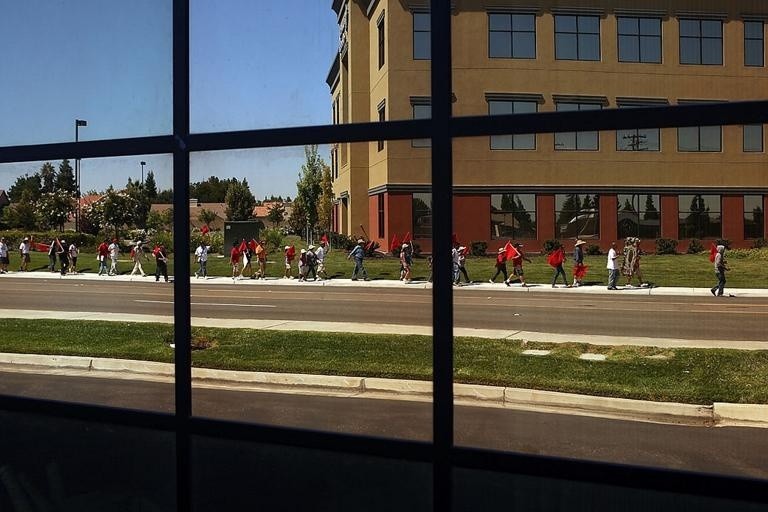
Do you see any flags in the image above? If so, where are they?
[502,243,516,260]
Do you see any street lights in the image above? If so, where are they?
[73,117,88,233]
[139,159,147,184]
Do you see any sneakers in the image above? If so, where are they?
[608,286,615,290]
[626,285,634,287]
[711,288,716,296]
[194,272,432,283]
[641,283,648,287]
[452,280,473,286]
[488,278,495,284]
[553,283,586,288]
[522,283,527,287]
[504,280,510,286]
[0,267,173,284]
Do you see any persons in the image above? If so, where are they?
[488,248,511,287]
[606,236,648,290]
[298,240,325,282]
[425,246,473,285]
[347,239,372,281]
[282,245,295,279]
[0,237,171,283]
[572,240,586,287]
[711,245,729,297]
[551,244,570,288]
[194,241,212,280]
[503,242,532,287]
[400,243,411,282]
[230,240,269,281]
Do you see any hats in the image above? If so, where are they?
[358,239,365,244]
[574,239,587,247]
[401,244,409,253]
[457,246,467,252]
[285,241,325,253]
[497,247,506,255]
